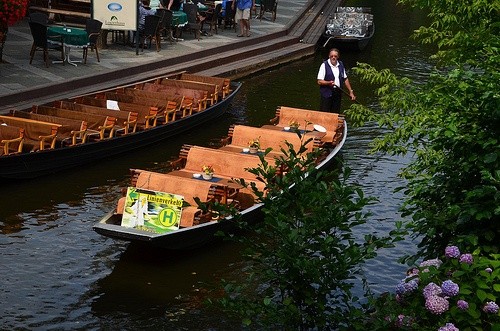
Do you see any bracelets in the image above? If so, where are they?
[349,89,353,93]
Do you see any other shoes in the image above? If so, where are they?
[246,32,251,37]
[237,33,244,37]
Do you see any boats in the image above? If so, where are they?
[0,73,244,183]
[93,106,348,248]
[321,7,375,60]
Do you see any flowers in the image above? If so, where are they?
[200,163,216,175]
[289,118,300,127]
[248,140,260,149]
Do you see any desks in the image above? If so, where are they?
[171,11,188,41]
[167,171,249,199]
[261,126,327,139]
[196,4,208,36]
[219,145,284,162]
[47,27,88,67]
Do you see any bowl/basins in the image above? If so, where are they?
[284,127,291,131]
[243,148,250,152]
[193,173,201,178]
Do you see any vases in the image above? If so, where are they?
[201,172,213,180]
[249,147,258,155]
[291,125,299,132]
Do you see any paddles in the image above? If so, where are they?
[335,84,361,104]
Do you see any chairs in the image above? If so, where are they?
[28,11,103,69]
[137,0,279,52]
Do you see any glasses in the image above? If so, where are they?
[330,55,338,58]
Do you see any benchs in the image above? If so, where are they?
[0,74,345,226]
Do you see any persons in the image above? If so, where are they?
[317,48,356,113]
[139,0,256,48]
